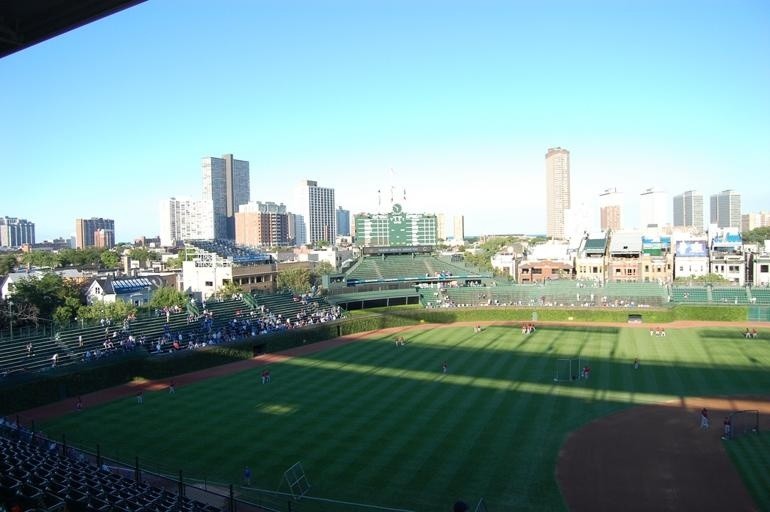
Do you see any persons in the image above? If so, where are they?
[701,408,711,427]
[661,326,665,336]
[583,366,592,378]
[634,357,641,371]
[0,296,224,443]
[424,269,651,307]
[478,323,482,332]
[395,336,398,348]
[751,328,760,339]
[521,321,536,334]
[241,463,250,485]
[655,325,660,337]
[442,360,450,373]
[650,325,654,336]
[743,327,752,339]
[723,415,731,441]
[401,336,405,347]
[261,368,271,384]
[224,284,342,342]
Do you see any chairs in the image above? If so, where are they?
[0,432,223,512]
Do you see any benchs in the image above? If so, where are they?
[342,253,494,281]
